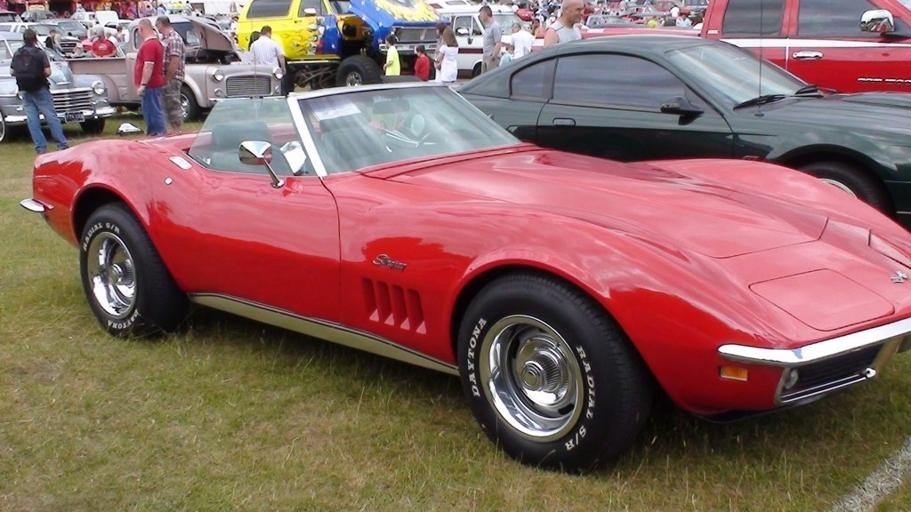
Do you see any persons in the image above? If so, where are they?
[383,0,708,84]
[10,29,70,154]
[155,15,186,132]
[134,19,169,136]
[250,26,287,96]
[46,0,239,59]
[246,31,260,63]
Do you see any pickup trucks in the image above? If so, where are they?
[59,10,269,125]
[579,1,909,95]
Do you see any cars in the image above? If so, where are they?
[453,33,881,210]
[1,34,112,140]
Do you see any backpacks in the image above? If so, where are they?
[14,48,45,92]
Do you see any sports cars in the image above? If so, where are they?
[19,83,911,476]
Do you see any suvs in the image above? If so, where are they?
[233,0,454,86]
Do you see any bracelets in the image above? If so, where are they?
[140,83,148,86]
